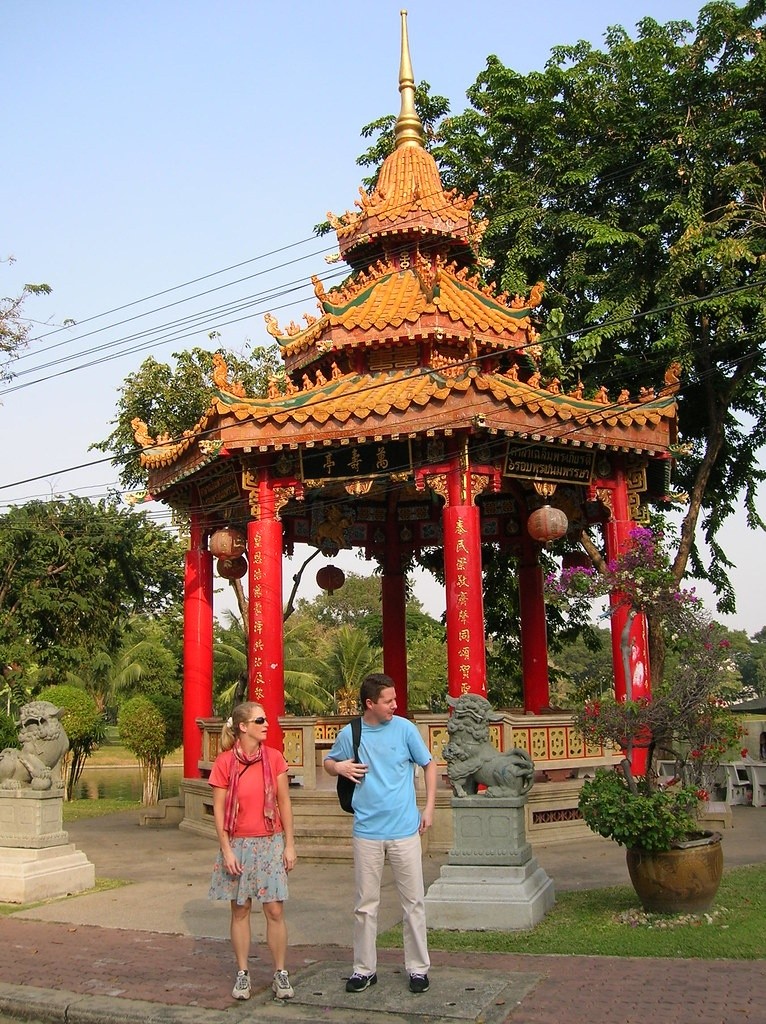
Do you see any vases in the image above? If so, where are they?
[620,830,724,914]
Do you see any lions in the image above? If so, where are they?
[0,699,71,791]
[441,692,536,798]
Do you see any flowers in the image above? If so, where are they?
[543,531,750,848]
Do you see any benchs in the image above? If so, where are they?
[314,738,337,767]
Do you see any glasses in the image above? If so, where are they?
[244,717,267,724]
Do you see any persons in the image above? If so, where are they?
[206,701,298,1000]
[323,673,436,993]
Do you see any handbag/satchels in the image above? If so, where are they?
[337,717,361,814]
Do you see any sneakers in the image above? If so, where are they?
[271,970,294,999]
[231,970,251,1000]
[410,974,430,992]
[346,972,377,992]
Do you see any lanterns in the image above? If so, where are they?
[528,507,566,546]
[217,556,247,586]
[210,529,245,568]
[562,551,591,569]
[316,565,345,596]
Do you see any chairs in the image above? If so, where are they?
[654,760,766,808]
